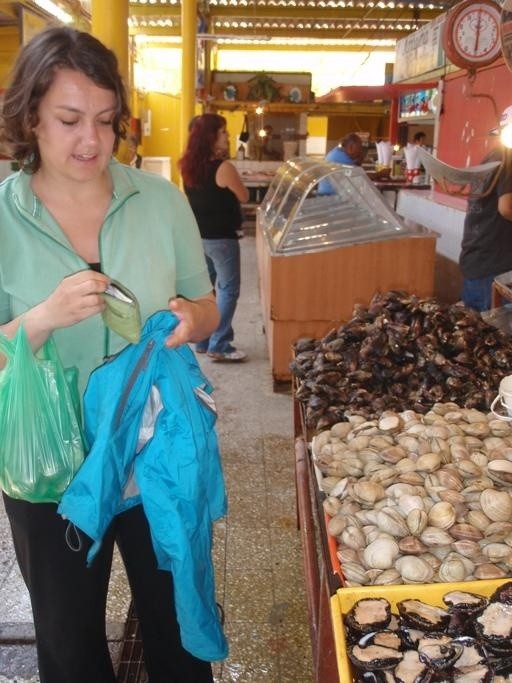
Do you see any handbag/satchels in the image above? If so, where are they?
[239,131,249,141]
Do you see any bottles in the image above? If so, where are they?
[399,102,430,118]
[236,143,245,160]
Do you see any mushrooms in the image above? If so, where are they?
[344,579,512,682]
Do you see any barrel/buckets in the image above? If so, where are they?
[490,374,512,427]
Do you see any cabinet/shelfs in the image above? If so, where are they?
[255,154,442,394]
[292,270,512,681]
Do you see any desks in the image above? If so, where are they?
[235,173,273,211]
[369,180,431,213]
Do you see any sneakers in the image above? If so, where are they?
[196,347,247,360]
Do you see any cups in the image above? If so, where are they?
[405,167,421,184]
[374,161,390,181]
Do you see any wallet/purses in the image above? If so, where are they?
[95,279,142,344]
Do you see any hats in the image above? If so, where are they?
[488,105,512,137]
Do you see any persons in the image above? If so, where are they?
[318,134,362,196]
[0,27,220,683]
[237,125,280,161]
[180,114,250,360]
[413,131,427,152]
[459,106,512,314]
